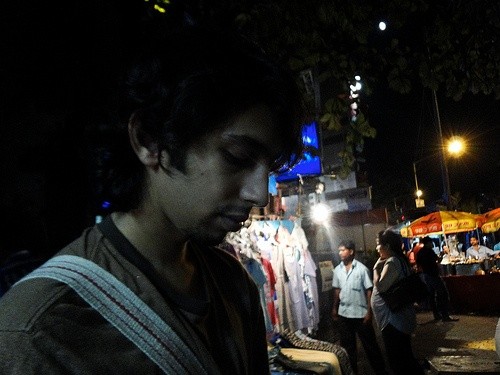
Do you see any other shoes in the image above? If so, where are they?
[432,315,460,322]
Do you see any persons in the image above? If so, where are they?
[0,49,304,375]
[332,228,459,375]
[465,237,500,261]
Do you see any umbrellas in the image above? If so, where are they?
[401,208,500,244]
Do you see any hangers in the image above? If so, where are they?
[226,215,300,235]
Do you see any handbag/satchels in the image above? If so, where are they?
[379,269,429,314]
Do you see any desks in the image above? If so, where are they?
[439,272,500,316]
